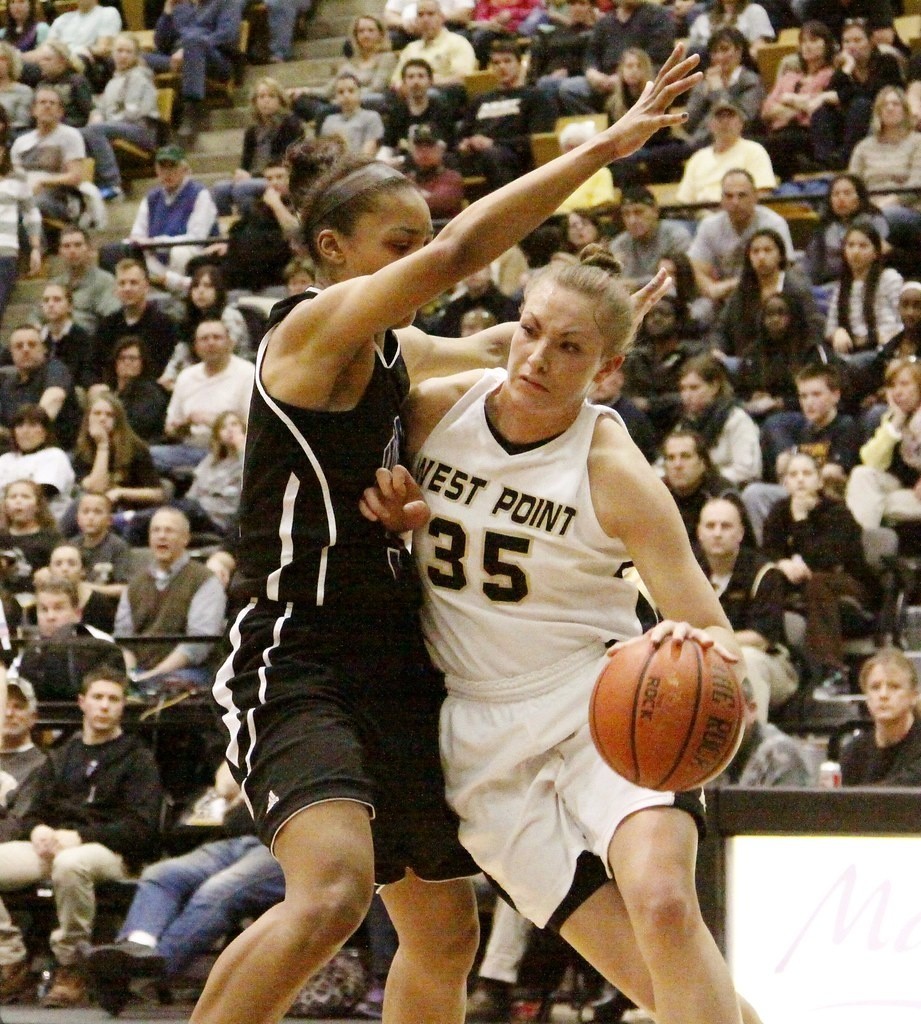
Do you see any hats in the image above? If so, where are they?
[3,673,36,703]
[709,94,742,114]
[47,39,70,60]
[414,124,439,147]
[156,145,185,162]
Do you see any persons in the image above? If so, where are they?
[0,0,921,1024]
[188,39,703,1023]
[0,668,163,1009]
[835,649,921,792]
[361,242,761,1024]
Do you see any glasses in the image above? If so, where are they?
[118,354,138,363]
[844,18,866,27]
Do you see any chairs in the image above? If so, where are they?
[39,156,96,230]
[106,87,174,177]
[154,19,250,108]
[247,3,308,37]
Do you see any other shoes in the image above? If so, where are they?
[1,942,644,1024]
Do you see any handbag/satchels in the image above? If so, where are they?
[293,946,364,1014]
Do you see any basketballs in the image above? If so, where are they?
[582,630,749,797]
